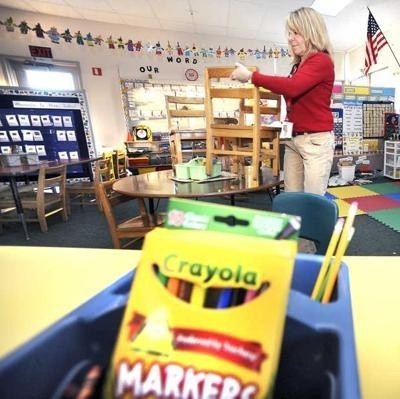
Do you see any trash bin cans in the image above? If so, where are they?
[337,163,356,181]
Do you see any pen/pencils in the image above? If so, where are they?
[108,199,358,399]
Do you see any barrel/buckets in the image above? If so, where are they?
[339,164,356,180]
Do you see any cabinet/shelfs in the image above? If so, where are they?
[383,140,400,180]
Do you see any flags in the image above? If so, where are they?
[364,13,388,76]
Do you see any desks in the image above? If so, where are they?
[0,244,400,399]
[112,165,284,215]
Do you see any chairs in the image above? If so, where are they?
[163,66,282,181]
[0,149,168,250]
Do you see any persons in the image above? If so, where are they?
[230,7,336,197]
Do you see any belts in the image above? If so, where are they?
[291,128,319,137]
